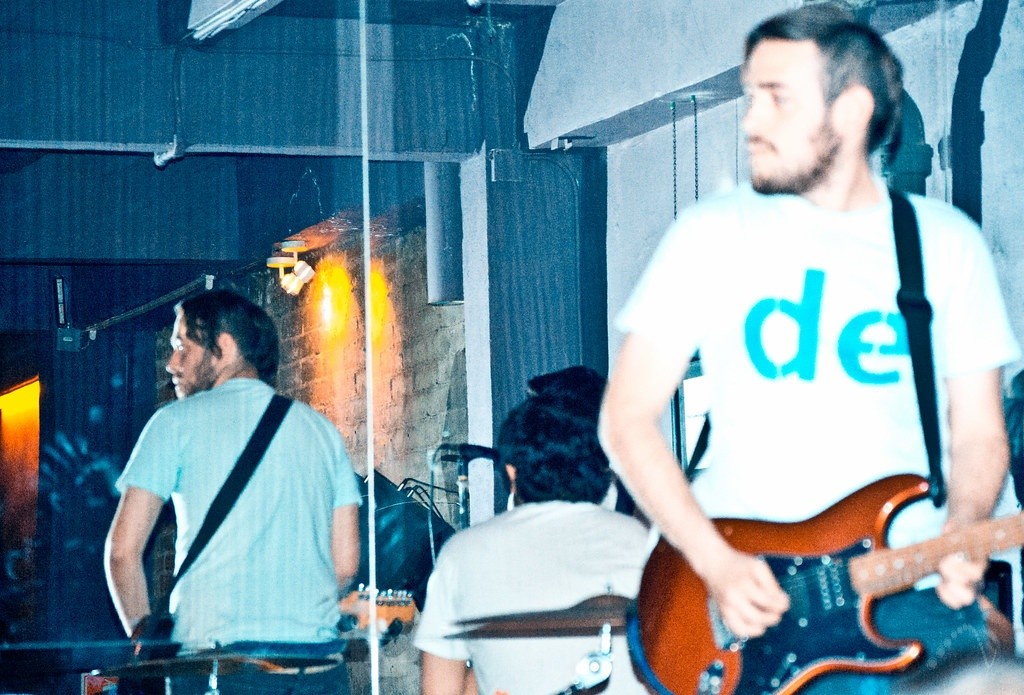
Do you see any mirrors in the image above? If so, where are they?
[0,145,494,695]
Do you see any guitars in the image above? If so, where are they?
[79,582,416,695]
[628,468,1024,695]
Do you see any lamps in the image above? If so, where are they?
[268,241,315,297]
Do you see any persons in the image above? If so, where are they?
[596,5,1023,695]
[103,286,364,695]
[414,365,658,695]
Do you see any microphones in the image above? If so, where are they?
[442,443,498,460]
